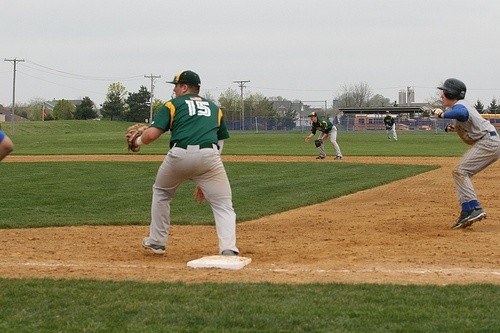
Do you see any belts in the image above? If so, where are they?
[175,142,213,149]
[480,130,497,139]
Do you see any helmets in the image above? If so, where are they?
[438,78,466,99]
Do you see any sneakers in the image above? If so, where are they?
[461,206,486,222]
[141,237,166,254]
[452,210,472,229]
[222,250,236,256]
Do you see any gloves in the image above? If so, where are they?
[431,108,444,119]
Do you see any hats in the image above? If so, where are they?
[308,111,317,117]
[386,111,389,113]
[166,70,201,87]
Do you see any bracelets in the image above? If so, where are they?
[136,136,144,146]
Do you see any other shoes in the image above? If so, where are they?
[335,156,342,159]
[316,156,325,160]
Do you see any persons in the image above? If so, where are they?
[126,71,241,256]
[302,111,344,160]
[383,116,398,142]
[430,77,500,229]
[0,130,14,159]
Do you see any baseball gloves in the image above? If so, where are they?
[385,123,392,130]
[444,123,457,133]
[126,123,149,153]
[314,139,324,148]
